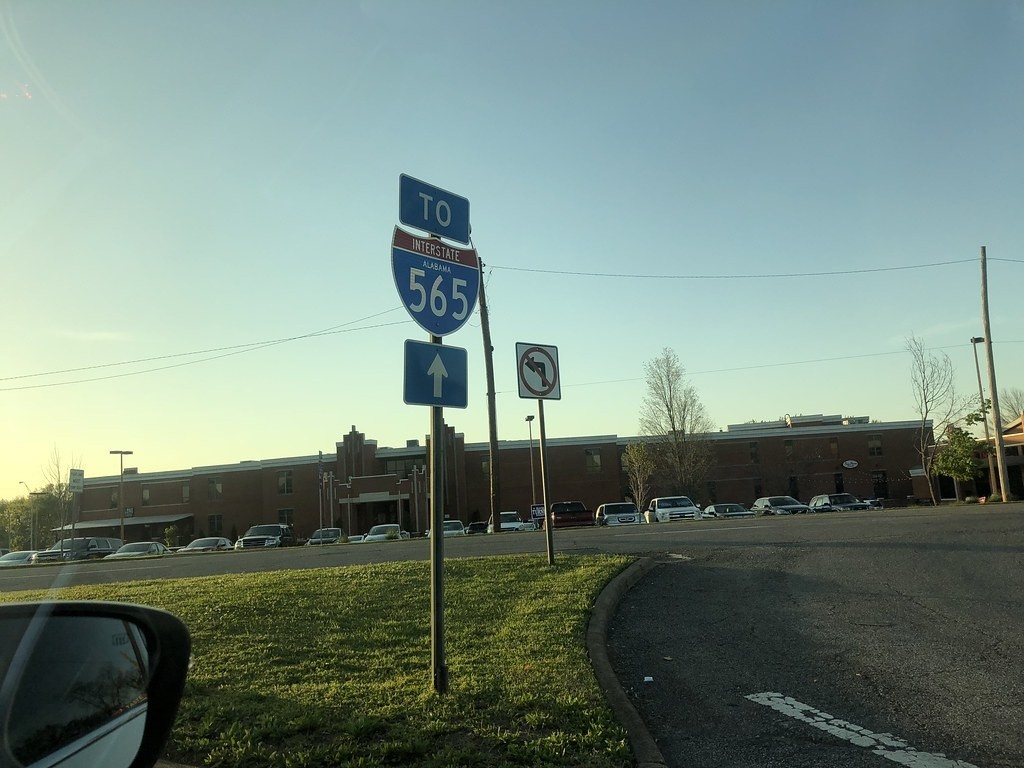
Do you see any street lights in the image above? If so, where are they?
[970,336,996,497]
[525,415,535,530]
[110,450,134,542]
[18,481,34,551]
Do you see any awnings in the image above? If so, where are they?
[50,513,192,531]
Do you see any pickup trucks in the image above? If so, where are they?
[541,501,596,530]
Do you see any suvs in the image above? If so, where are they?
[642,496,703,523]
[233,523,298,550]
[30,537,125,564]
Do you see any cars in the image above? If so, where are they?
[176,537,234,552]
[307,528,348,545]
[102,542,172,559]
[347,535,365,544]
[522,523,537,531]
[424,519,467,538]
[594,502,647,526]
[483,511,528,534]
[808,493,872,513]
[0,551,42,566]
[702,504,756,519]
[857,499,884,510]
[747,495,811,517]
[362,524,407,541]
[465,521,487,534]
